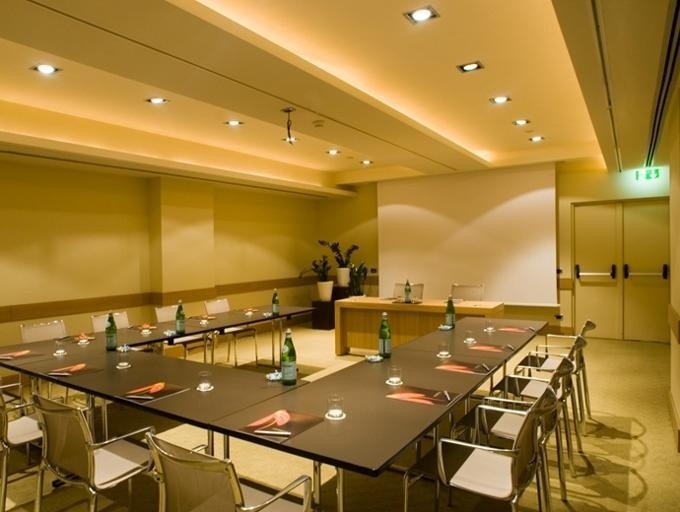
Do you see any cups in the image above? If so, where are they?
[484,318,496,330]
[77,331,89,343]
[438,342,450,356]
[117,352,132,369]
[141,326,151,335]
[326,393,345,419]
[464,328,475,342]
[197,369,211,389]
[55,341,64,354]
[199,319,208,328]
[387,365,402,383]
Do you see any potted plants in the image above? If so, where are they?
[311,256,335,301]
[347,260,368,299]
[319,240,359,286]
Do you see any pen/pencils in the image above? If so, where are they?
[507,344,517,351]
[529,327,535,331]
[126,396,154,399]
[482,363,491,372]
[0,356,14,360]
[129,324,134,330]
[253,430,292,436]
[444,390,451,401]
[57,334,70,342]
[48,372,72,376]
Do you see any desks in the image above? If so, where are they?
[2,304,316,370]
[332,293,506,358]
[13,348,309,462]
[206,317,550,512]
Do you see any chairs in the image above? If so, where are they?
[28,388,160,512]
[204,296,259,368]
[18,318,69,399]
[447,283,487,301]
[400,386,550,512]
[1,381,66,512]
[392,282,425,301]
[153,305,202,360]
[449,319,595,500]
[145,431,315,511]
[90,310,130,333]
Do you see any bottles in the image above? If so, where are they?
[404,279,411,304]
[271,287,279,315]
[378,312,391,359]
[104,312,118,350]
[174,299,185,335]
[280,328,297,387]
[445,295,455,328]
